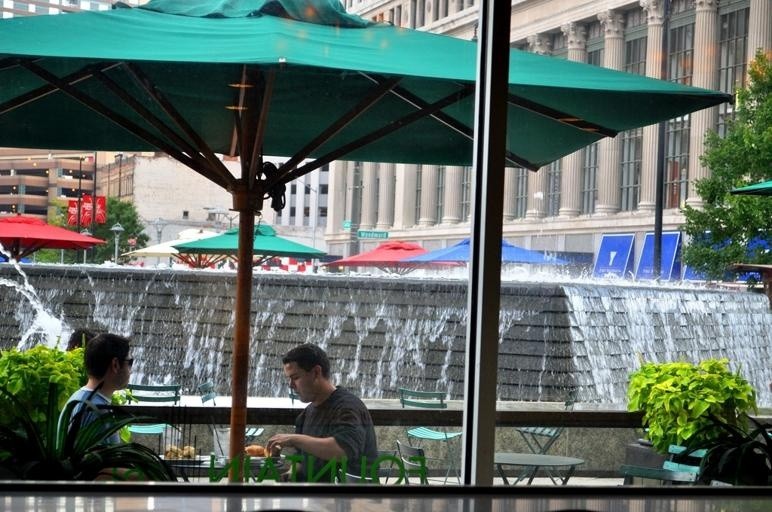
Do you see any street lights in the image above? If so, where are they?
[80,229,94,264]
[77,155,85,233]
[114,153,123,198]
[111,222,125,266]
[153,217,167,266]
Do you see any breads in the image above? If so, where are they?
[265,446,281,457]
[183,446,196,458]
[246,446,264,455]
[165,446,181,459]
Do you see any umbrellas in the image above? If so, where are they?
[320,237,465,280]
[0,1,737,510]
[0,214,105,267]
[117,225,232,269]
[397,235,570,273]
[171,220,329,272]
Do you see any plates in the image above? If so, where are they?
[158,456,205,466]
[242,453,286,461]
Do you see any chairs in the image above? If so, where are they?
[514,386,579,485]
[126,381,183,455]
[197,377,265,455]
[397,385,464,484]
[337,468,376,485]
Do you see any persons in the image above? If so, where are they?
[51,334,151,482]
[261,340,379,482]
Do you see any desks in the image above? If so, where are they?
[494,453,586,487]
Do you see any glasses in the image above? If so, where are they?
[123,359,134,367]
